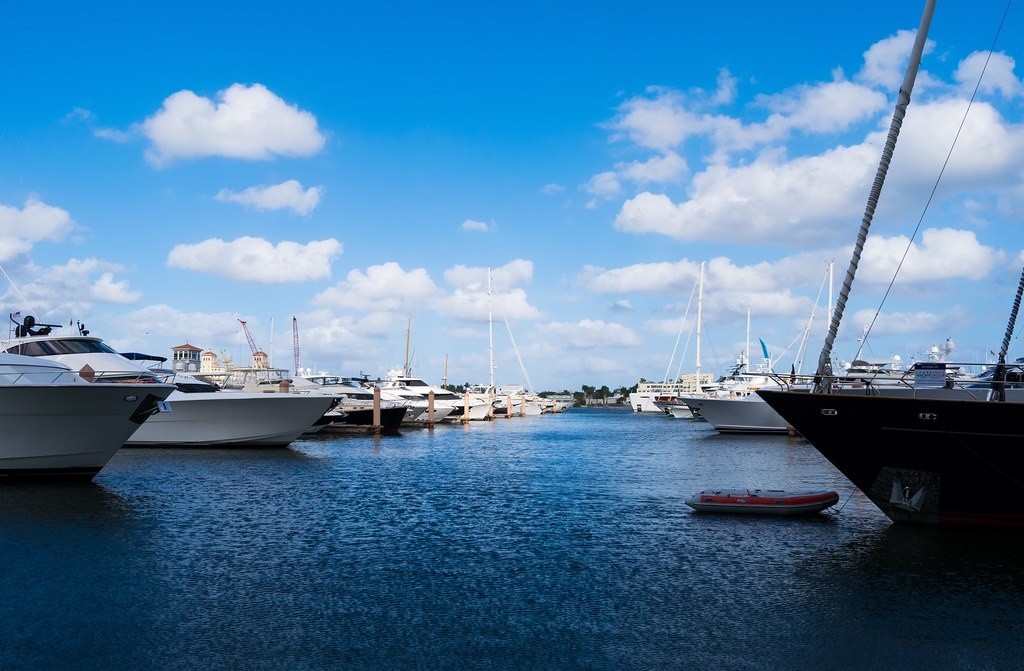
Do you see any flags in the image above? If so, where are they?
[759,338,771,361]
[990,350,995,357]
[12,312,21,318]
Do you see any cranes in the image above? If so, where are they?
[292,316,302,379]
[233,317,270,377]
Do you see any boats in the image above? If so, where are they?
[651,261,789,419]
[674,259,1024,435]
[220,366,575,433]
[755,1,1024,556]
[122,352,344,449]
[0,266,178,481]
[684,485,842,516]
